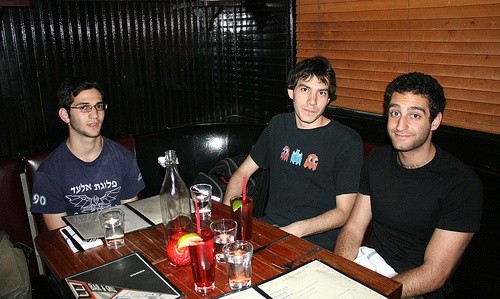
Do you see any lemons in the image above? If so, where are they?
[178,233,203,247]
[233,200,242,212]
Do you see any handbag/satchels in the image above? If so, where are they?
[0,229,32,299]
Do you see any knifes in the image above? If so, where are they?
[253,233,292,254]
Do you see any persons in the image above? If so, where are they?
[0,230,63,299]
[333,72,483,299]
[30,78,146,230]
[222,55,365,253]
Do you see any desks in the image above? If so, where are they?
[33,191,402,299]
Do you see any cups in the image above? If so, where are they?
[189,226,216,297]
[222,240,253,290]
[190,184,213,221]
[230,195,252,241]
[100,208,125,249]
[210,219,237,264]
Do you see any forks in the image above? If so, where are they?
[272,247,322,270]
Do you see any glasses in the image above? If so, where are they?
[70,103,108,111]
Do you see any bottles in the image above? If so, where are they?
[159,150,195,267]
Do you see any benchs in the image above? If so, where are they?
[1,124,500,299]
